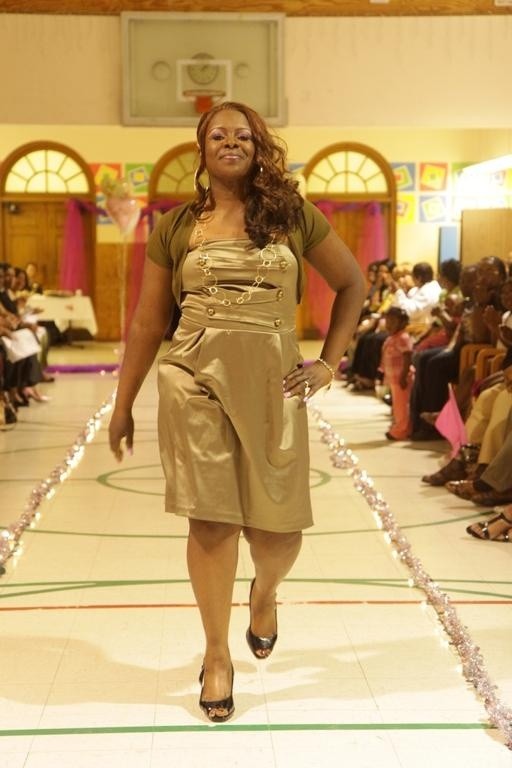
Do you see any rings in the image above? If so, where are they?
[304,380,310,396]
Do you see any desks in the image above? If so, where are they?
[18,292,97,349]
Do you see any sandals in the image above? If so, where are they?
[198,654,236,723]
[493,529,512,542]
[466,512,511,540]
[246,575,277,659]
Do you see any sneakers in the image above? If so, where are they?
[349,381,376,394]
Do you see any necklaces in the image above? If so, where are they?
[191,211,276,311]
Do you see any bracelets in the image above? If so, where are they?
[317,357,334,390]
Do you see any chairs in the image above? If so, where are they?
[458,342,507,389]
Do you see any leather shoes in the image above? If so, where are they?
[422,475,430,482]
[430,459,466,484]
[445,479,477,500]
[41,376,54,382]
[472,487,512,505]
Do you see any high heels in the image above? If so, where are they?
[12,398,29,412]
[22,386,51,402]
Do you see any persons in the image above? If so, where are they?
[1,259,59,433]
[105,100,368,722]
[342,257,512,542]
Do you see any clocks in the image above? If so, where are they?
[189,54,219,84]
